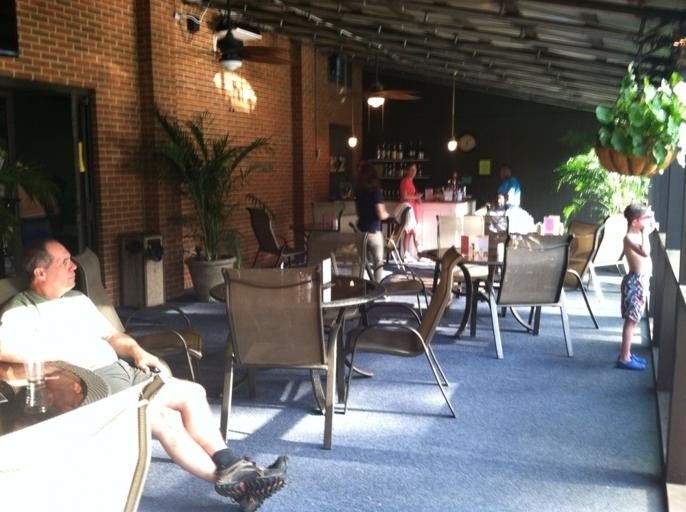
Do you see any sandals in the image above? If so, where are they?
[617,353,647,370]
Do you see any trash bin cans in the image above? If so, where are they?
[118,231,165,308]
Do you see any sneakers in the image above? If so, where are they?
[214,455,290,511]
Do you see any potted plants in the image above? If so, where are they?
[121,105,277,304]
[592,59,686,176]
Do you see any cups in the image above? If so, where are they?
[297,270,313,289]
[298,290,312,304]
[24,347,45,383]
[25,383,47,415]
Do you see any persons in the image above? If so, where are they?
[398,163,424,262]
[617,204,652,371]
[496,164,522,208]
[354,163,390,283]
[0,238,289,511]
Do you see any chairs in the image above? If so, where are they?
[0,275,31,312]
[70,246,205,382]
[0,372,166,512]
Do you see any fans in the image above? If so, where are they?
[333,52,425,103]
[185,0,291,66]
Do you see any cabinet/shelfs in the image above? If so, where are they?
[366,158,433,200]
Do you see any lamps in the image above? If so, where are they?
[218,59,244,112]
[366,97,386,134]
[215,21,263,42]
[447,74,459,152]
[347,95,358,149]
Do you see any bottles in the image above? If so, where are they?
[375,139,426,198]
[434,171,472,200]
[329,153,347,172]
